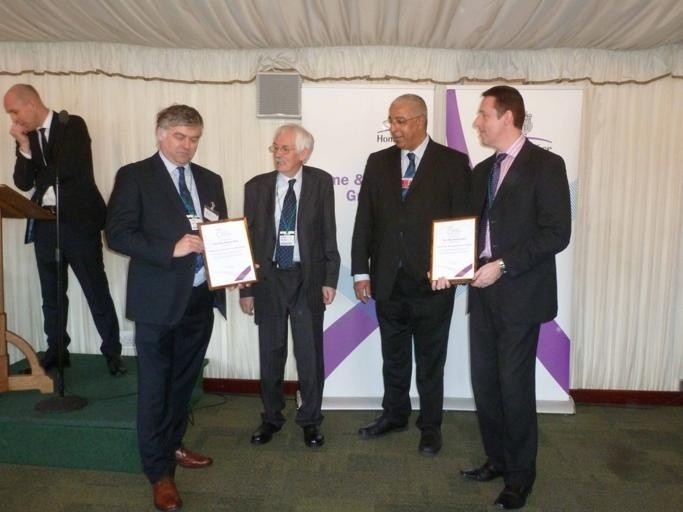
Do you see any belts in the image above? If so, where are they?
[41,206,60,215]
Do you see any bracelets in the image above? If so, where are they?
[496,255,508,275]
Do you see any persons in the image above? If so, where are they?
[239,122,340,448]
[349,92,472,457]
[3,83,128,380]
[97,104,228,510]
[428,85,573,508]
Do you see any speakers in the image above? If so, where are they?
[257,71,301,119]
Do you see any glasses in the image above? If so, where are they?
[383,116,420,126]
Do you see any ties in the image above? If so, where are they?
[175,166,205,273]
[400,152,417,205]
[38,127,62,205]
[474,154,509,260]
[275,179,298,271]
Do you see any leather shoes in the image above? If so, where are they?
[359,413,409,439]
[175,446,212,468]
[460,457,502,482]
[419,424,443,456]
[40,346,71,368]
[104,354,130,376]
[153,477,183,511]
[250,421,281,445]
[494,483,533,510]
[303,424,324,448]
